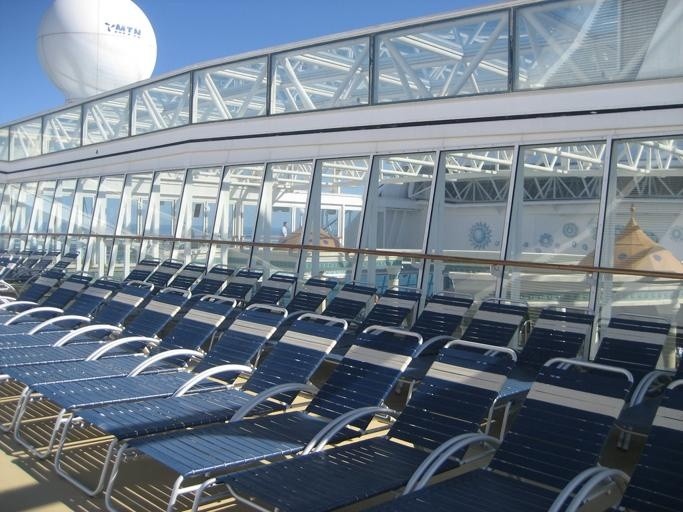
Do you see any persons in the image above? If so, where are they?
[281,221,289,239]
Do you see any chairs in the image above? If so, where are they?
[1,249,681,510]
